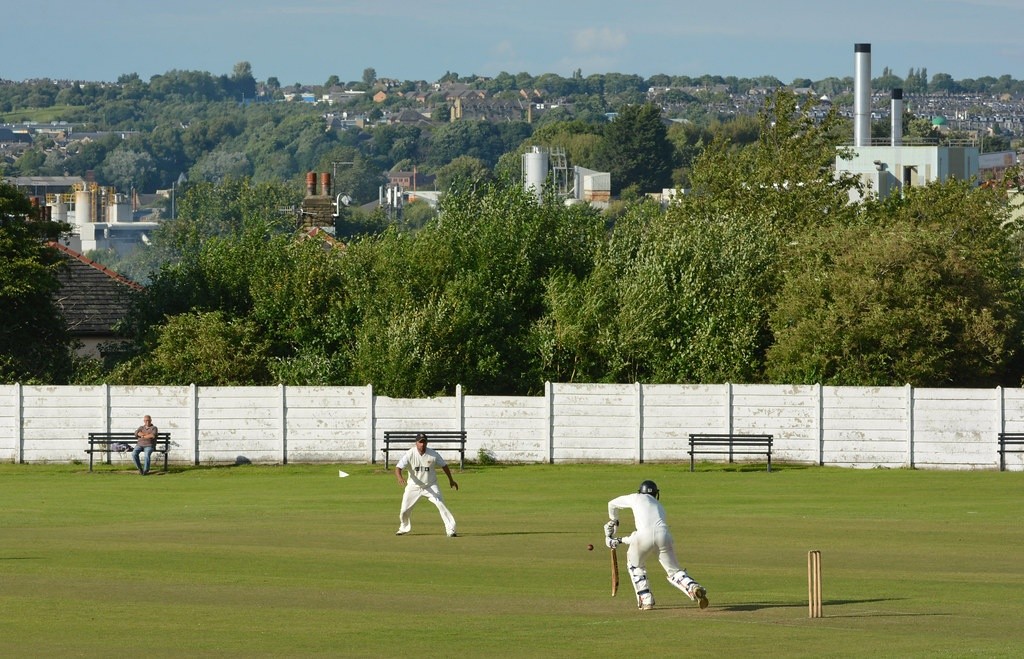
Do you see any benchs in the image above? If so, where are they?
[380,431,468,469]
[84,433,172,471]
[997,433,1024,471]
[687,433,774,472]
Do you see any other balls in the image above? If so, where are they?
[587,544,594,550]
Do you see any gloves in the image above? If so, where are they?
[610,538,621,548]
[604,522,615,537]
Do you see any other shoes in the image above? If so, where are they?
[142,471,146,475]
[447,533,456,538]
[138,469,143,475]
[396,531,406,535]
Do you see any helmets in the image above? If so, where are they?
[639,481,657,498]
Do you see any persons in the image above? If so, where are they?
[604,480,709,610]
[395,433,459,537]
[132,415,158,476]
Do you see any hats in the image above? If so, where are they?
[416,434,427,442]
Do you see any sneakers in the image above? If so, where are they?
[639,604,653,610]
[693,586,709,610]
